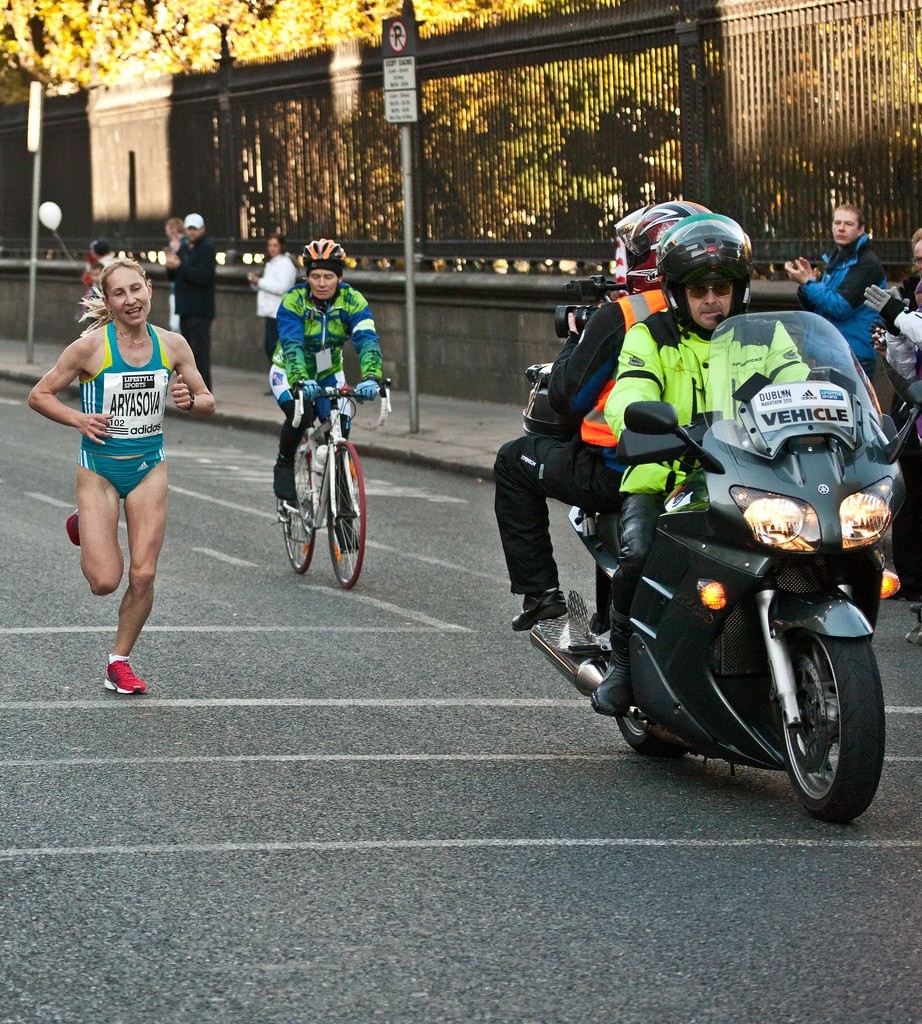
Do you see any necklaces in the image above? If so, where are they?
[118,330,149,344]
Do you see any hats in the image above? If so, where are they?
[185,213,204,229]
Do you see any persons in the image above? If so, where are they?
[81,240,113,331]
[248,235,299,397]
[267,239,382,550]
[28,258,216,694]
[162,212,217,396]
[493,198,850,716]
[863,228,922,645]
[784,205,887,391]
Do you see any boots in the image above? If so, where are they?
[591,604,633,717]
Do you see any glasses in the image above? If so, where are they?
[685,281,731,298]
[912,256,920,265]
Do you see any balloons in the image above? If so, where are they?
[39,201,62,230]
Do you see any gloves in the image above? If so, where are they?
[294,379,321,400]
[863,285,910,314]
[869,319,888,345]
[356,380,379,405]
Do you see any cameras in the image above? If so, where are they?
[878,331,888,346]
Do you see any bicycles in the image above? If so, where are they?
[275,376,393,590]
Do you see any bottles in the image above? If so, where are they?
[314,445,327,488]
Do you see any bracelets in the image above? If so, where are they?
[185,392,194,410]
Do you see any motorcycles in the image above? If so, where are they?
[525,309,921,826]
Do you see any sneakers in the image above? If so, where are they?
[274,454,297,501]
[512,588,567,631]
[65,509,80,546]
[104,660,147,694]
[335,518,360,550]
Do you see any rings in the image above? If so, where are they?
[180,390,182,396]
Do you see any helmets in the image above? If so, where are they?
[625,200,713,295]
[302,238,347,276]
[656,213,752,325]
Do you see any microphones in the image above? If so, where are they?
[562,280,605,300]
[716,314,727,324]
